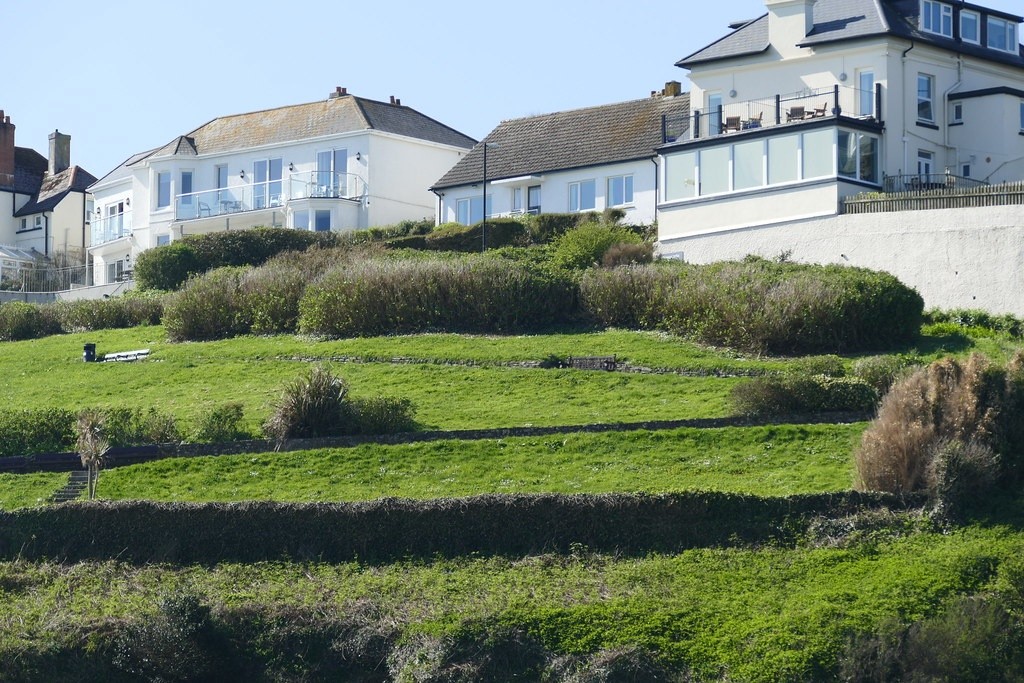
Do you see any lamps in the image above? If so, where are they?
[356,152,360,160]
[288,163,293,170]
[240,170,244,178]
[96,207,100,214]
[126,198,130,205]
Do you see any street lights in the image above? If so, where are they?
[479,141,500,251]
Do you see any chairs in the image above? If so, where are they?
[786,103,827,124]
[198,201,211,217]
[721,111,763,133]
[229,201,241,212]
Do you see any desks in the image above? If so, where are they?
[217,199,233,214]
